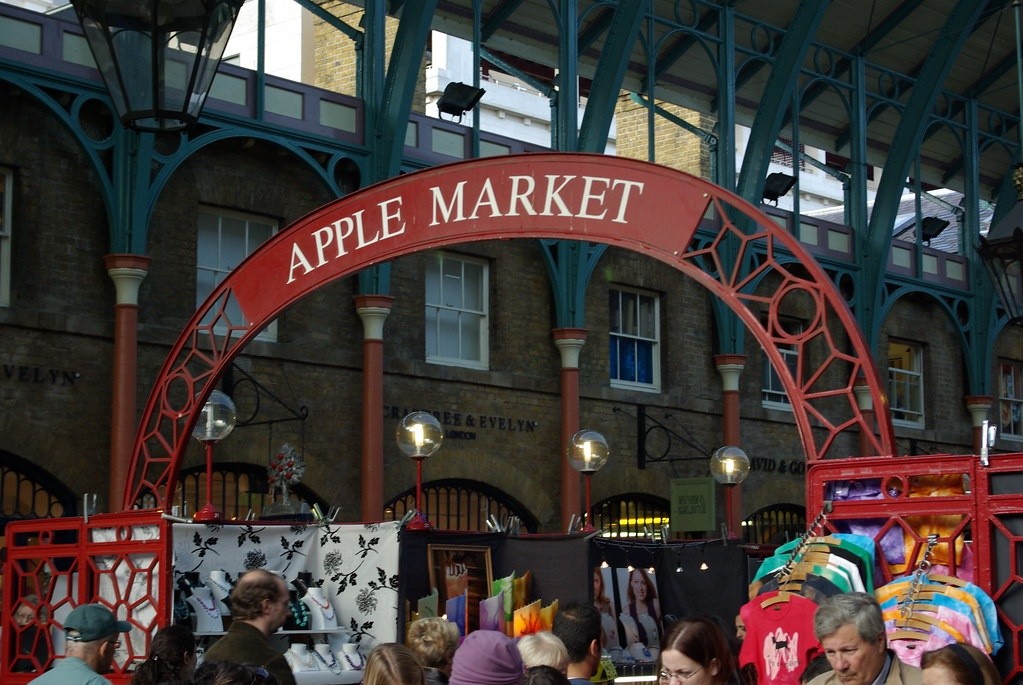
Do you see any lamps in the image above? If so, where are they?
[913,216,951,249]
[599,540,709,574]
[437,81,486,126]
[762,173,799,208]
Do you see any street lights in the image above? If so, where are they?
[710,446,750,539]
[183,389,237,522]
[394,411,444,530]
[566,429,609,533]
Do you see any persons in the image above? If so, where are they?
[132,626,197,685]
[193,569,297,685]
[620,569,661,623]
[593,568,615,622]
[920,643,1001,685]
[735,613,757,685]
[799,593,921,685]
[655,616,741,685]
[363,600,604,685]
[27,603,132,685]
[11,594,54,673]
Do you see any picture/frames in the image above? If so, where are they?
[427,544,493,631]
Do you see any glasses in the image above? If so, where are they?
[100,640,121,648]
[191,650,203,658]
[660,665,704,682]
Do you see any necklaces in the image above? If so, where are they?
[288,648,315,668]
[289,598,307,626]
[602,614,615,639]
[298,579,317,591]
[313,650,343,675]
[312,596,335,620]
[345,651,364,670]
[641,615,657,639]
[174,600,190,620]
[620,618,638,643]
[194,596,220,619]
[208,577,232,594]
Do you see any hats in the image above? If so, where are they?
[64,604,133,642]
[449,630,522,685]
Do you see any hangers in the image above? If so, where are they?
[888,551,973,645]
[778,507,841,597]
